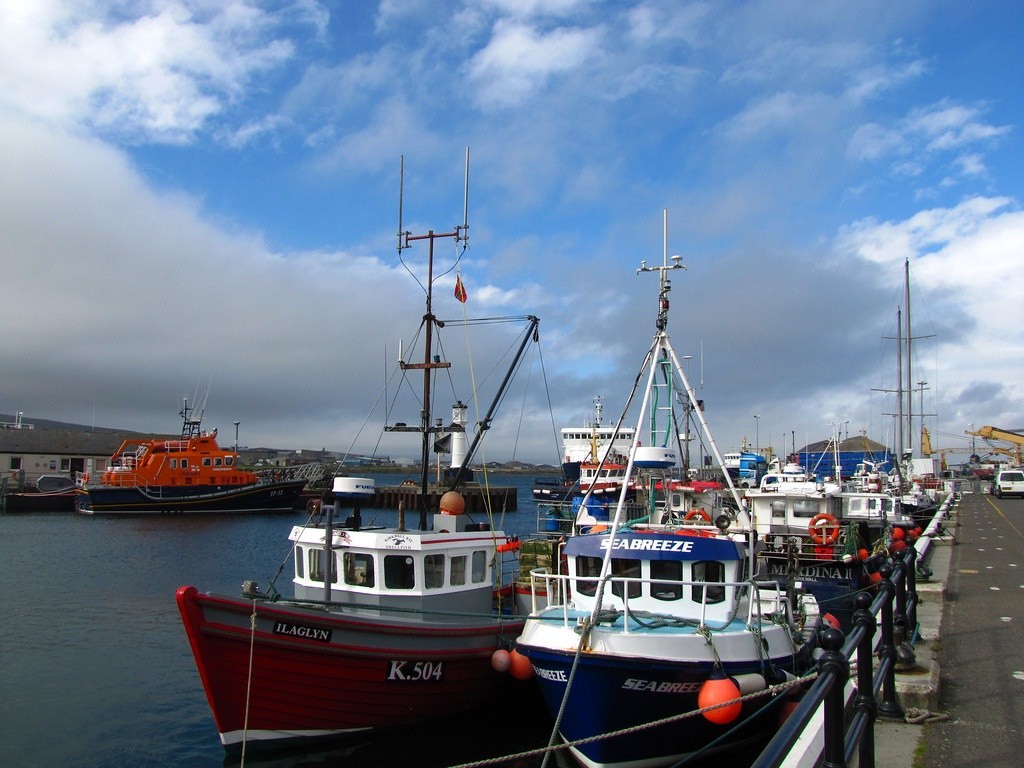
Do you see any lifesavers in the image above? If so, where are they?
[83,472,89,484]
[809,514,840,544]
[684,509,711,525]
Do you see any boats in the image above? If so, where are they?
[73,398,322,517]
[510,204,826,768]
[533,391,945,558]
[174,141,553,762]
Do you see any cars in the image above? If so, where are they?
[990,471,1024,499]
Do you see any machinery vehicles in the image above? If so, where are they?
[964,426,1024,464]
